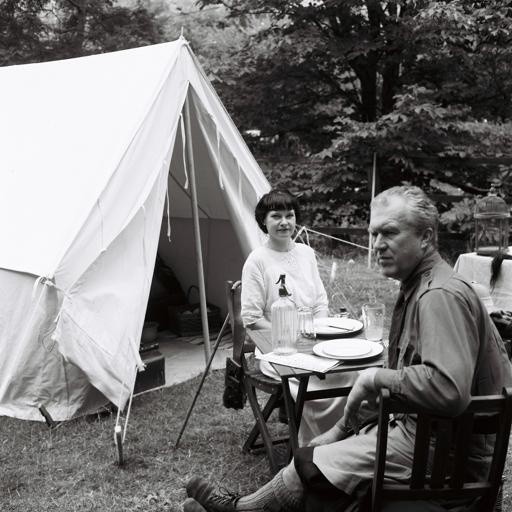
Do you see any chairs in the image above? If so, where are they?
[293,384,512,511]
[225,277,286,476]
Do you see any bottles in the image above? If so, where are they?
[270,273,299,356]
[335,307,352,318]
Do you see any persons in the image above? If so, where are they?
[240,188,363,450]
[180,184,511,512]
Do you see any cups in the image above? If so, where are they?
[361,303,386,341]
[297,307,317,346]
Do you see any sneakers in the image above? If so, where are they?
[273,421,292,469]
[181,474,246,512]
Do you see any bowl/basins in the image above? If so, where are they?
[140,322,159,343]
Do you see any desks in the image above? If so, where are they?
[458,246,512,317]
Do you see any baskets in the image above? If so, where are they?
[168,285,222,337]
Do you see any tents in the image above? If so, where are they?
[0,36,273,421]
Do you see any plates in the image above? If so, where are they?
[301,318,384,362]
